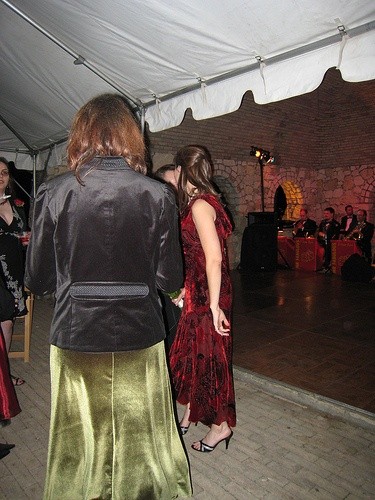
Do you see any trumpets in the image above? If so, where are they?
[292,218,304,236]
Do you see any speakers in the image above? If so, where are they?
[247,211,278,272]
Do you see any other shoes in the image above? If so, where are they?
[0,443,15,460]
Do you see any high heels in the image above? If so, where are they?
[179,421,199,435]
[191,430,233,452]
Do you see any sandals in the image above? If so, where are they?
[10,374,25,386]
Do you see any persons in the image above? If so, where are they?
[293,208,317,238]
[167,145,237,451]
[25,94,193,497]
[1,157,27,386]
[318,208,340,268]
[153,162,182,361]
[356,209,373,263]
[340,205,357,239]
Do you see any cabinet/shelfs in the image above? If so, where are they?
[293,238,325,273]
[331,240,362,275]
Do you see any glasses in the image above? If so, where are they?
[0,169,10,177]
[300,214,305,217]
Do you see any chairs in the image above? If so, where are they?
[8,286,34,363]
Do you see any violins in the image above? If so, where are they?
[346,221,367,238]
